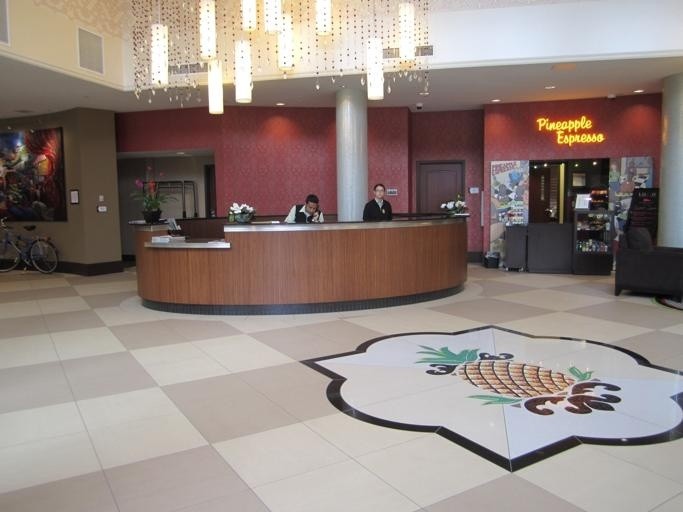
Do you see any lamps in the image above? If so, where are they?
[131,0,429,114]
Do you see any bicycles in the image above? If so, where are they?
[0,216,59,273]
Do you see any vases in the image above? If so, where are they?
[234,213,253,224]
[141,211,161,223]
[447,211,456,218]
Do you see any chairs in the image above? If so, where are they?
[616,234,683,296]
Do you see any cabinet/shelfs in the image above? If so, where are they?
[572,209,614,274]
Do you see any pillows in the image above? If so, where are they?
[629,226,653,248]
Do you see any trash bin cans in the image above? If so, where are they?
[484,251,500,268]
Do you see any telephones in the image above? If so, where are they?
[307,208,318,222]
[152,235,173,244]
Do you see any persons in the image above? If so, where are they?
[285,194,325,223]
[363,184,393,222]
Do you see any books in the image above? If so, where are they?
[151,234,186,243]
[208,239,225,244]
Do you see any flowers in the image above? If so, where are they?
[229,202,256,215]
[440,200,467,210]
[129,163,178,210]
